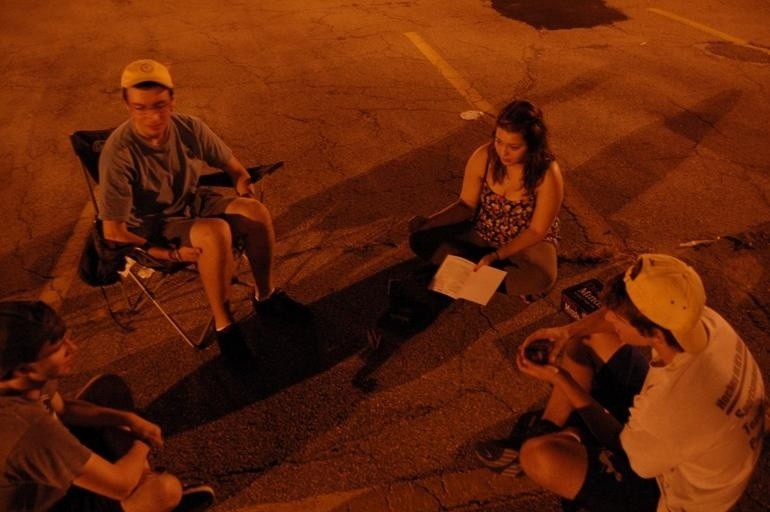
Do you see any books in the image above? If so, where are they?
[426,253,508,305]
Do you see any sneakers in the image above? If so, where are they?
[251,287,312,320]
[471,431,539,480]
[182,480,216,511]
[214,322,250,380]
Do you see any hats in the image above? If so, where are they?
[120,59,174,91]
[623,253,709,354]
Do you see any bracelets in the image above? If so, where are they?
[166,248,182,264]
[495,251,500,260]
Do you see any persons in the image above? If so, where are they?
[0,298,218,510]
[95,58,316,382]
[408,96,567,298]
[517,248,766,510]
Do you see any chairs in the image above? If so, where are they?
[69,128,286,353]
